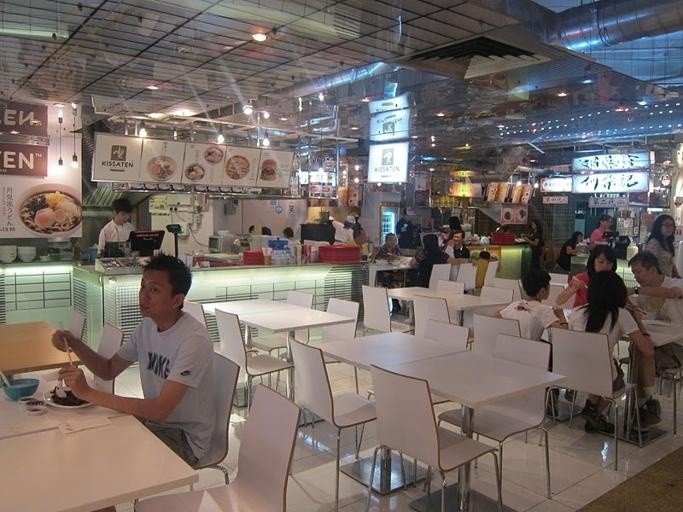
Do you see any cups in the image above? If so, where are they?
[263,255,272,265]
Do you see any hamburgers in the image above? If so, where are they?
[261,159,278,180]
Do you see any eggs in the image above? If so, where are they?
[54,209,66,222]
[34,208,56,227]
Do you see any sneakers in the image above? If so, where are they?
[580,398,616,434]
[565,389,577,404]
[546,388,560,417]
[630,395,664,433]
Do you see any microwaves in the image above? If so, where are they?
[207,235,234,253]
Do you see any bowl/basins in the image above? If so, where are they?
[39,255,48,261]
[0,245,37,264]
[145,146,250,181]
[3,378,39,399]
[14,184,82,236]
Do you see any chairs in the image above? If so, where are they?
[0,215,683,511]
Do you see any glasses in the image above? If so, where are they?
[660,222,675,228]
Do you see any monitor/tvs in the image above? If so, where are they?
[129,230,165,257]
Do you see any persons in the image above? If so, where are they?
[96,196,135,259]
[52,250,217,512]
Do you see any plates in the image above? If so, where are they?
[18,396,46,415]
[502,207,527,223]
[43,388,95,408]
[647,321,676,331]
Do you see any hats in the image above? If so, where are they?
[346,215,356,224]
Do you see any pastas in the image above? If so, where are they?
[21,192,83,231]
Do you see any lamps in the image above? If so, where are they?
[56,117,63,166]
[262,127,270,147]
[137,119,147,137]
[217,102,224,144]
[71,107,78,163]
